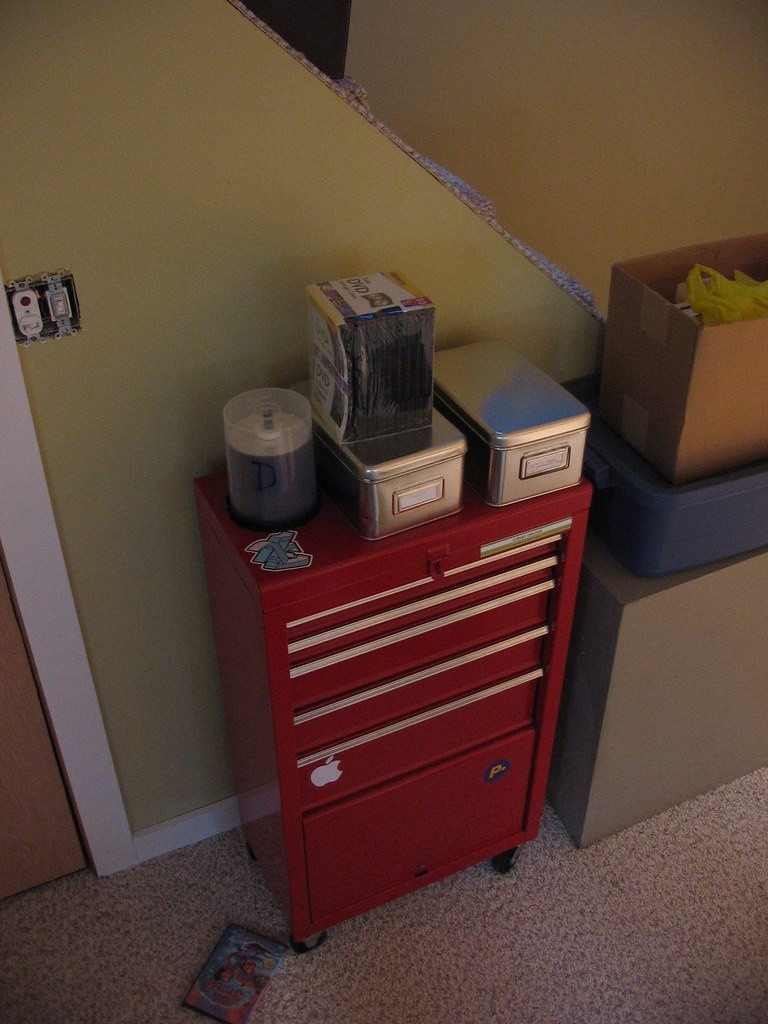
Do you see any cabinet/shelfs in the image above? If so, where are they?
[192,465,591,957]
[547,526,768,849]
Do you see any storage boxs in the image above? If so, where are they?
[562,372,768,578]
[601,232,768,485]
[308,407,466,541]
[304,269,436,447]
[435,337,589,506]
